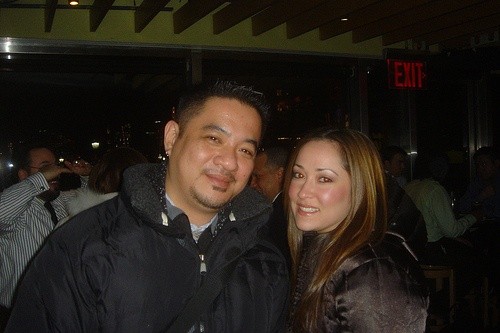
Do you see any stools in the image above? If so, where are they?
[407,264,456,333]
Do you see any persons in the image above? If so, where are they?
[281,126,430,333]
[54,145,149,228]
[402,140,485,321]
[455,146,500,282]
[249,146,295,237]
[380,145,410,224]
[0,140,95,332]
[7,80,291,333]
